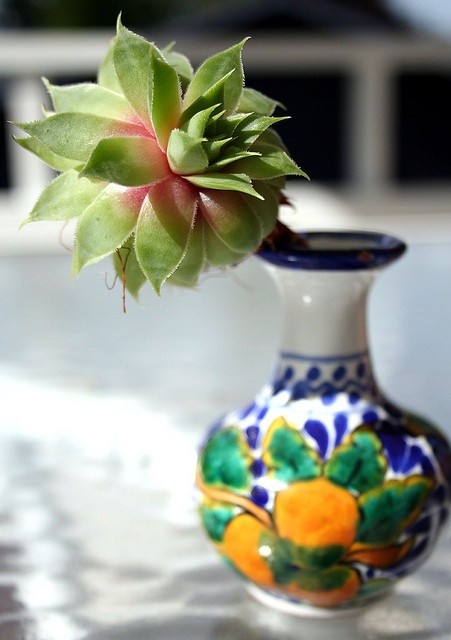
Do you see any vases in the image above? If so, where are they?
[194,228,451,619]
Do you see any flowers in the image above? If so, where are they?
[5,11,314,292]
